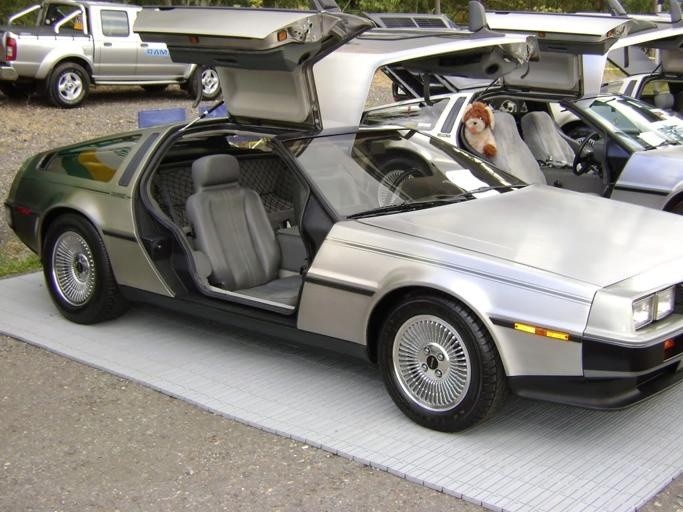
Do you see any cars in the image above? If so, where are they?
[1,0,221,109]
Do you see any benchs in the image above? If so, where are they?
[149,158,296,236]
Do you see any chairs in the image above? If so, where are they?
[654,93,683,120]
[490,111,549,187]
[186,154,304,307]
[520,110,583,174]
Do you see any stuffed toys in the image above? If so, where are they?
[461,100,496,159]
